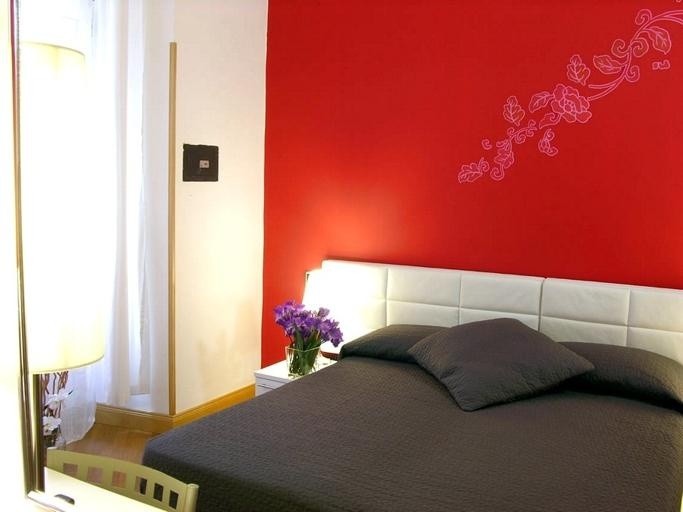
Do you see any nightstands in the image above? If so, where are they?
[255,357,338,397]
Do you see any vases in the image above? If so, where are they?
[283,344,321,375]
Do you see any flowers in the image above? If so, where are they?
[272,300,343,375]
[43,388,73,448]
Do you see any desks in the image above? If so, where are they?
[43,464,168,512]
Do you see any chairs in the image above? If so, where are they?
[45,447,199,512]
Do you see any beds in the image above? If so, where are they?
[139,260,683,512]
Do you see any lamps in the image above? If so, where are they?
[18,43,109,505]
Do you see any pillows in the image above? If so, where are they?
[406,319,595,411]
[557,330,682,417]
[338,324,451,364]
